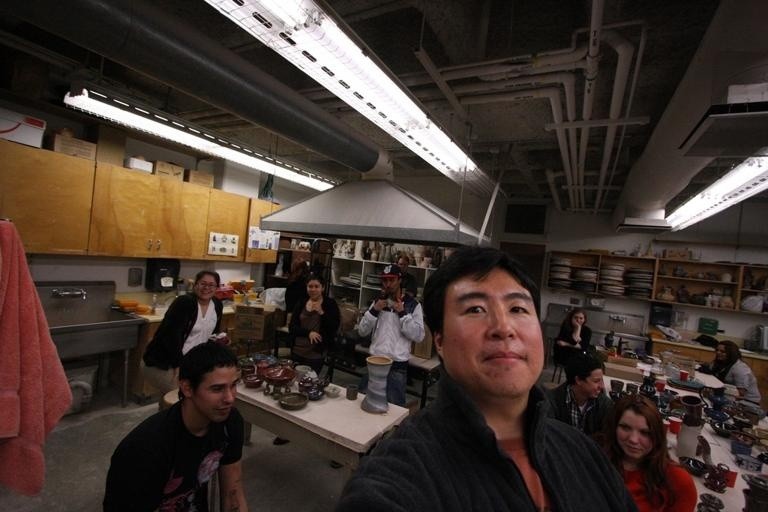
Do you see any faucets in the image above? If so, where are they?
[80,288,88,304]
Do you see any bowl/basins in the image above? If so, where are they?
[703,400,768,473]
[610,380,638,393]
[243,374,263,387]
[679,456,705,477]
[324,386,341,397]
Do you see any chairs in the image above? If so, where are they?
[550,336,565,384]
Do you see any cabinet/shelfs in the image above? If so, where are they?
[88,161,210,260]
[0,139,96,255]
[329,257,437,310]
[547,250,768,315]
[203,188,282,264]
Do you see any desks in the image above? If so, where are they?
[233,379,409,480]
[603,358,768,512]
[274,327,442,409]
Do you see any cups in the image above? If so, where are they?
[680,370,688,380]
[241,366,255,375]
[656,382,664,391]
[669,416,681,431]
[726,469,738,487]
[347,384,359,400]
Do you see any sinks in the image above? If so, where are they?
[541,302,649,353]
[33,281,140,361]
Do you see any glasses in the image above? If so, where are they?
[196,281,218,289]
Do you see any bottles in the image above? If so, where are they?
[657,263,736,309]
[360,355,394,413]
[332,237,443,268]
[289,237,314,252]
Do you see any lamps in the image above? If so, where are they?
[64,88,342,192]
[205,0,506,200]
[665,157,768,232]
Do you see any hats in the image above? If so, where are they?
[379,264,404,279]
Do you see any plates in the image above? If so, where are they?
[295,364,311,372]
[551,253,654,299]
[280,393,308,409]
[264,367,295,383]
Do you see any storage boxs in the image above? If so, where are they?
[0,109,214,188]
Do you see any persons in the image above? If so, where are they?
[546,353,612,437]
[101,342,249,511]
[600,390,700,511]
[140,269,225,410]
[331,245,638,510]
[555,307,594,381]
[273,234,426,470]
[706,341,763,406]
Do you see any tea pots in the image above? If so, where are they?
[299,377,313,391]
